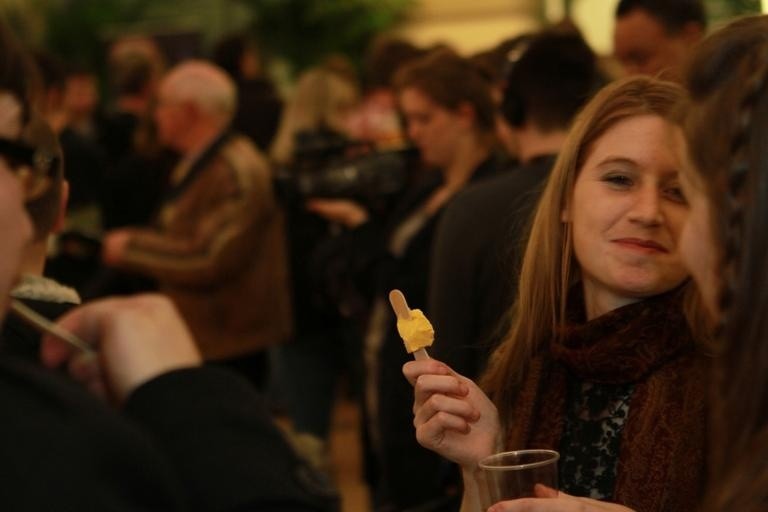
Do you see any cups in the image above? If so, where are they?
[476,448,561,512]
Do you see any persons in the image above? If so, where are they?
[2,0,768,512]
